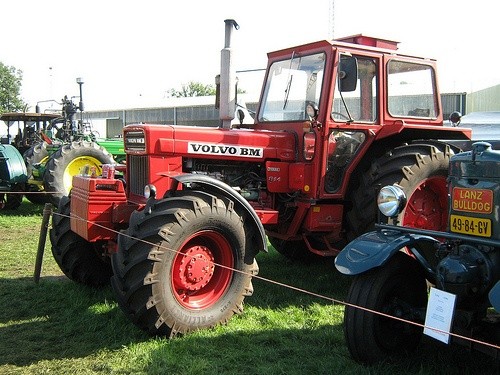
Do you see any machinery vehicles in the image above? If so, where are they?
[48,19,475,336]
[333,140,500,362]
[1,78,115,213]
